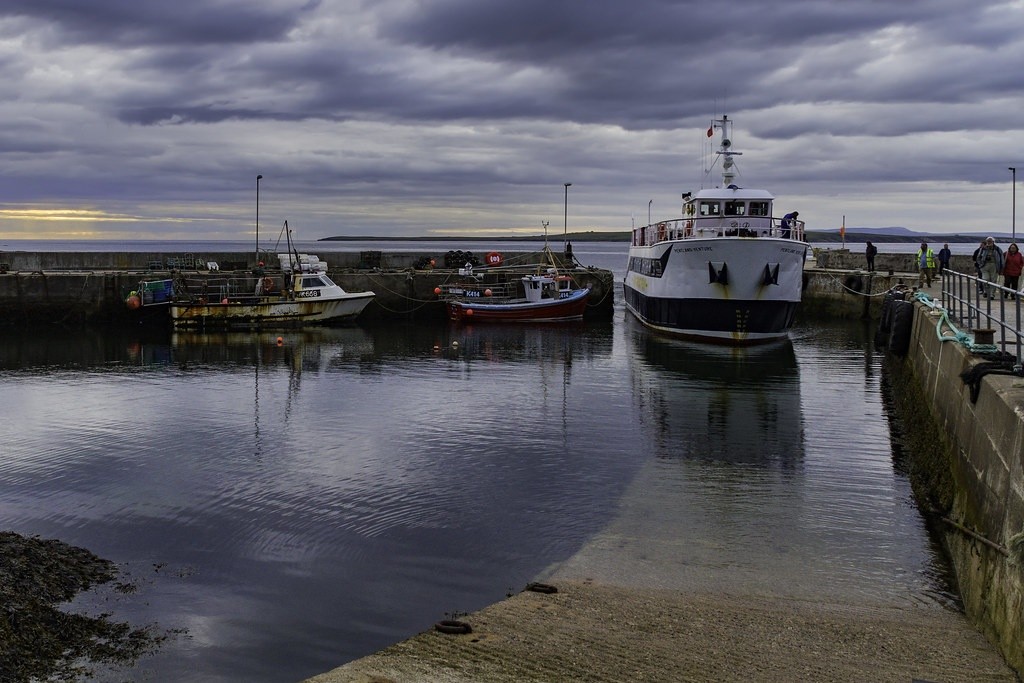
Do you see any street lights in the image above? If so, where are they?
[562,181,574,247]
[1007,166,1017,244]
[256,174,267,268]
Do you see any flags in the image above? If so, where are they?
[707,127,713,137]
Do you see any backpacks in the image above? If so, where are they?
[872,245,877,256]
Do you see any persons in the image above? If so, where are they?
[972,240,987,294]
[916,243,938,288]
[938,243,951,276]
[866,240,877,272]
[999,243,1024,300]
[252,262,267,293]
[976,236,1005,300]
[780,211,799,238]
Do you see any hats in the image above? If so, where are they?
[866,240,872,244]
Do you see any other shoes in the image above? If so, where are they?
[990,295,994,300]
[927,283,931,288]
[984,292,988,297]
[918,285,922,288]
[979,290,983,294]
[1004,293,1008,298]
[1011,296,1014,300]
[932,275,939,279]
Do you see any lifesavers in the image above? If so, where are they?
[888,300,914,354]
[884,290,904,327]
[802,274,808,292]
[845,276,862,294]
[262,277,274,291]
[878,294,896,334]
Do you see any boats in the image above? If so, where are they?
[441,221,596,326]
[620,113,812,355]
[121,220,376,327]
